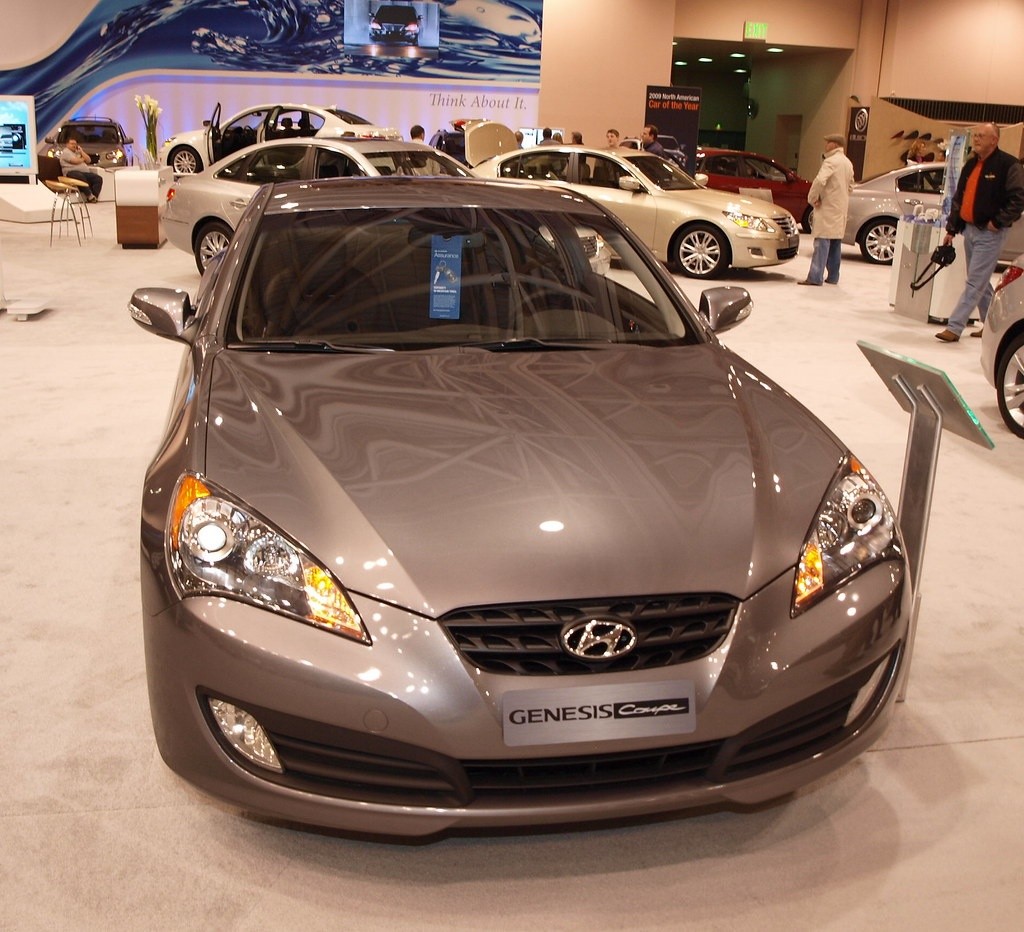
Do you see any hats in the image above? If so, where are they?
[573,131,583,141]
[823,133,846,148]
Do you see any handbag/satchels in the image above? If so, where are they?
[931,240,956,267]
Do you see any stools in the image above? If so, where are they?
[46,175,93,246]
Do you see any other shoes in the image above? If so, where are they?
[935,329,959,342]
[797,279,821,286]
[88,193,99,203]
[970,328,983,337]
[825,278,838,284]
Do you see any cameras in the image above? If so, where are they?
[931,245,955,267]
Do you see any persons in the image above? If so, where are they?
[60,139,102,202]
[797,135,854,286]
[899,140,941,193]
[402,125,441,177]
[935,122,1024,343]
[514,124,674,186]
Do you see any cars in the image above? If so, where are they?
[465,122,800,280]
[37,116,135,177]
[980,254,1024,439]
[0,132,24,152]
[368,4,423,46]
[840,158,1024,266]
[128,177,914,837]
[428,129,473,169]
[165,103,404,173]
[161,138,612,279]
[694,146,815,235]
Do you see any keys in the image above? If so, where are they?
[434,263,457,285]
